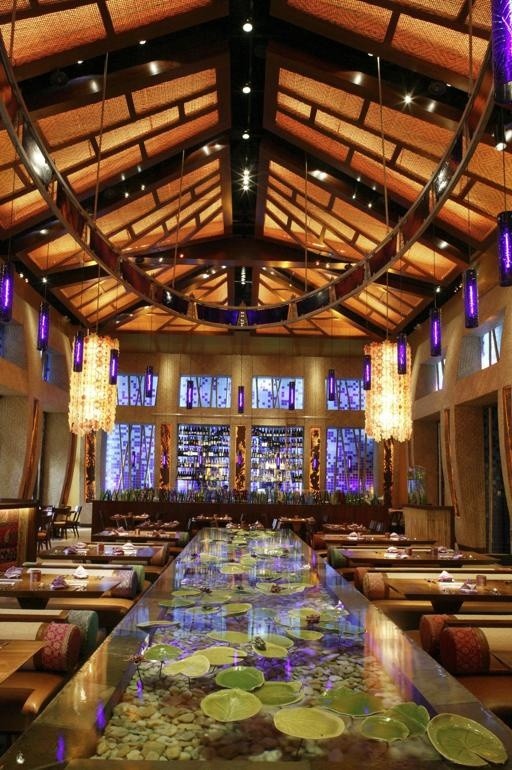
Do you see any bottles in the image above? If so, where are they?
[176,425,304,496]
[100,488,366,505]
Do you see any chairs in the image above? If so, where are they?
[261,513,270,529]
[240,512,246,526]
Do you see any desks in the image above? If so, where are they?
[394,505,454,550]
[0,506,233,770]
[388,508,404,534]
[272,517,512,729]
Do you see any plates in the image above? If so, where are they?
[73,548,92,552]
[435,580,464,586]
[2,575,24,583]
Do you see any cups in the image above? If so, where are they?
[476,575,487,586]
[30,571,40,581]
[98,543,105,553]
